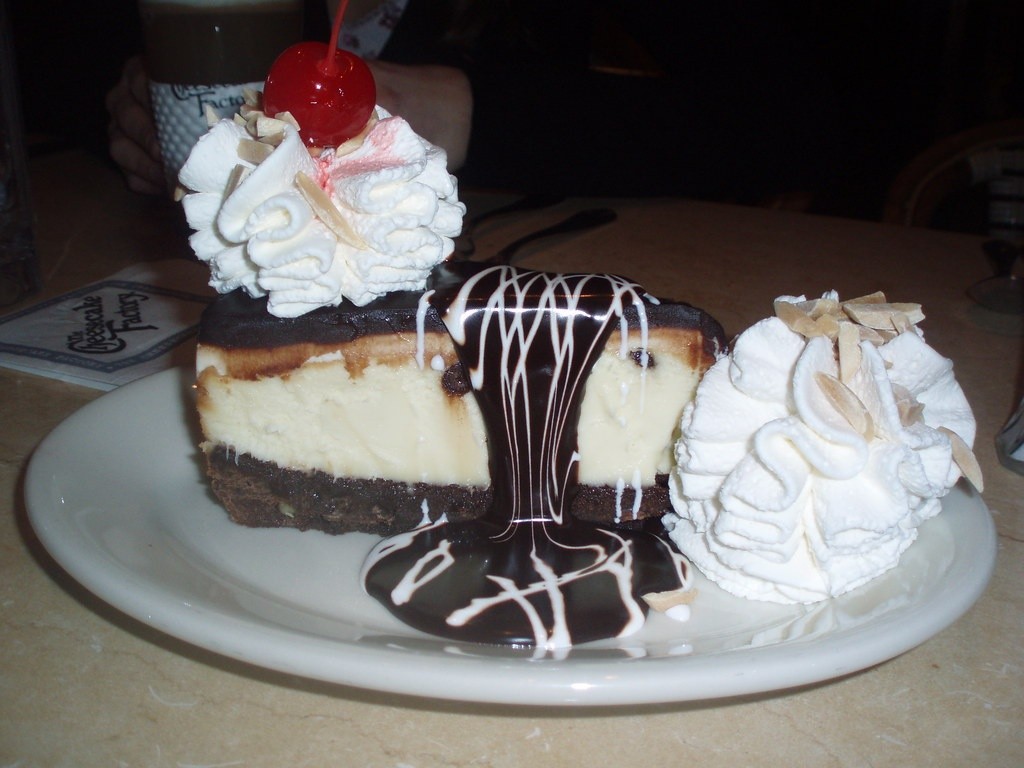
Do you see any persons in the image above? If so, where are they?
[106,0,603,202]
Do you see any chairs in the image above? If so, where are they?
[883,118,1024,231]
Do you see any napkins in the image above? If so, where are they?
[0,255,215,393]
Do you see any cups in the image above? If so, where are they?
[136,0,304,214]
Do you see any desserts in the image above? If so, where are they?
[176,0,983,649]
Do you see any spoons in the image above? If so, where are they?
[428,205,618,293]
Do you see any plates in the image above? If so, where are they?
[19,357,996,706]
